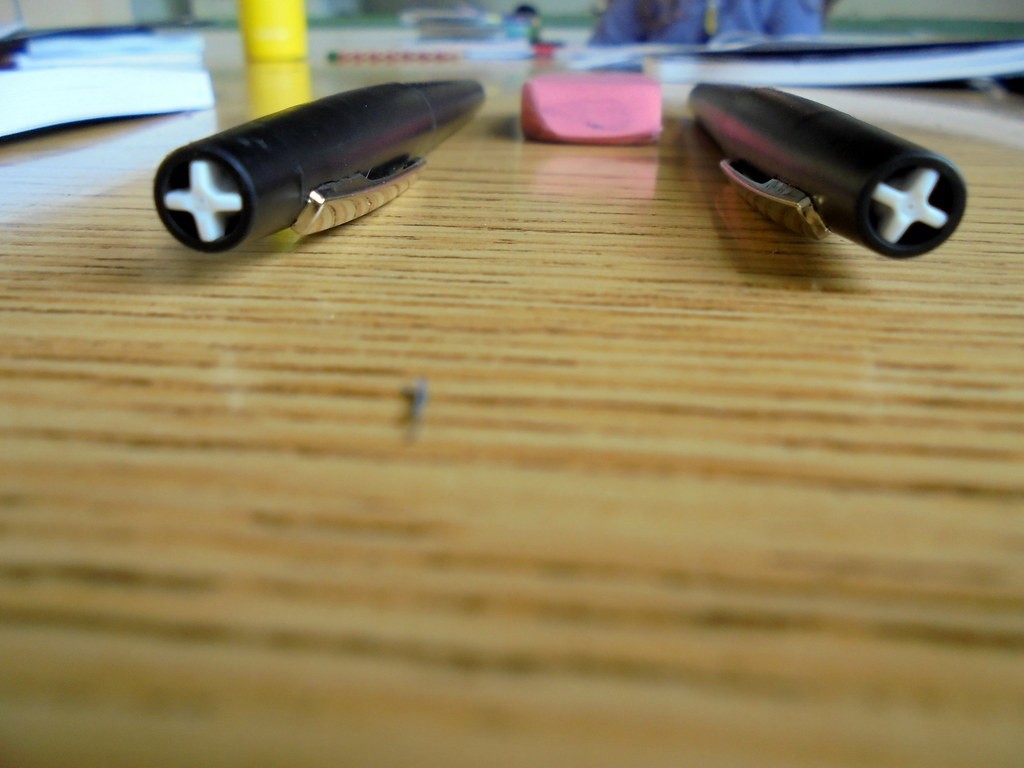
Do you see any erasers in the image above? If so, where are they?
[521,72,663,145]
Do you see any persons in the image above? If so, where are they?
[494,2,544,44]
[591,0,832,70]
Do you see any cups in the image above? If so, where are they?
[238,0,308,62]
[244,64,310,120]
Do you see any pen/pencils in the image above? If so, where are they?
[685,81,969,260]
[150,76,488,255]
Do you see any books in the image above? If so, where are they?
[0,21,216,138]
[642,35,1024,88]
